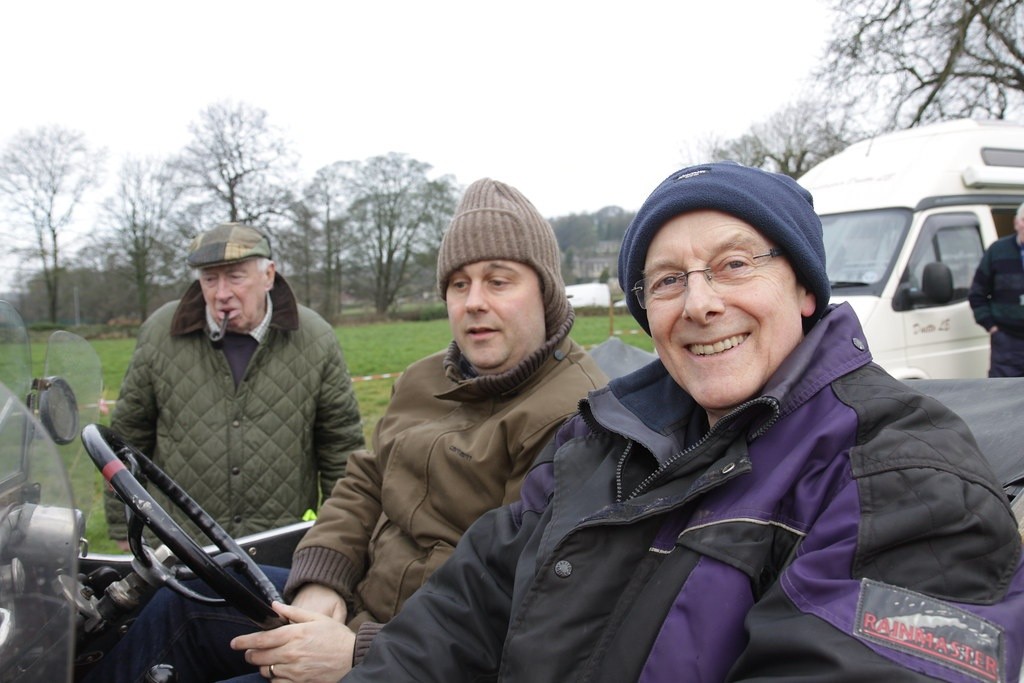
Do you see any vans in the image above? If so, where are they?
[566,283,611,309]
[794,120,1024,385]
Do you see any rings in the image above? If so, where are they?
[269,665,276,678]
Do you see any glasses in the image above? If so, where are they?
[632,246,786,307]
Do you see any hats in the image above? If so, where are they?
[618,161,830,334]
[188,222,271,268]
[435,177,570,338]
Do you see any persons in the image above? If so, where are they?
[102,225,363,555]
[88,175,612,683]
[344,162,1024,683]
[969,203,1024,379]
